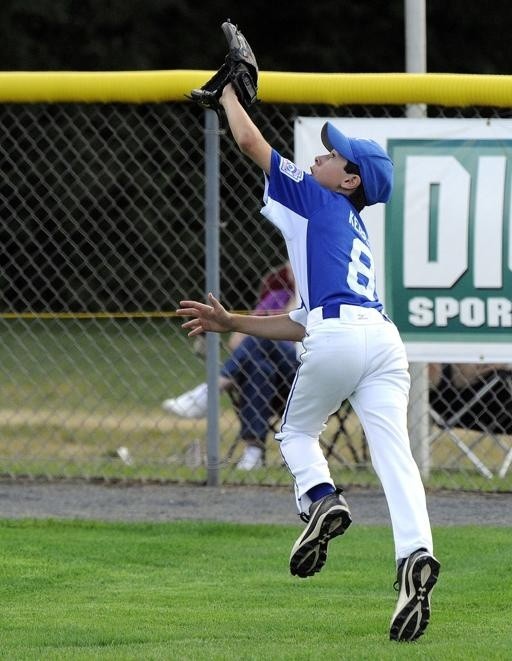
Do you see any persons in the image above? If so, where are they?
[176,18,441,642]
[158,259,300,474]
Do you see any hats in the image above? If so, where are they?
[321,123,393,204]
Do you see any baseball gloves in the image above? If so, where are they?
[190,22,259,118]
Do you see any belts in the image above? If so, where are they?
[322,306,390,323]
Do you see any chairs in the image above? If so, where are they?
[221,362,511,479]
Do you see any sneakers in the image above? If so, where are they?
[234,446,263,473]
[162,385,208,418]
[290,495,352,577]
[390,547,439,641]
